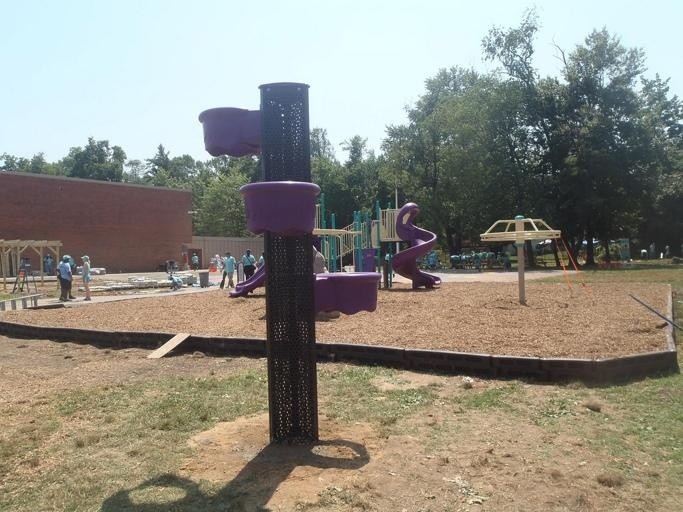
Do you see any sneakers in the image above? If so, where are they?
[83,298,92,301]
[60,296,76,302]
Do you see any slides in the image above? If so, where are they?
[229,265,265,298]
[392,202,441,289]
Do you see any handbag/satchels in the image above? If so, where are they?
[252,263,256,270]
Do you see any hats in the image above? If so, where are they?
[81,256,89,260]
[63,255,71,260]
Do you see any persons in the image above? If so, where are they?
[258,252,264,267]
[243,248,255,293]
[80,255,91,300]
[223,252,236,288]
[168,275,183,291]
[211,253,227,268]
[385,251,395,278]
[192,253,199,272]
[183,254,189,271]
[639,240,670,258]
[44,253,54,276]
[56,253,76,300]
